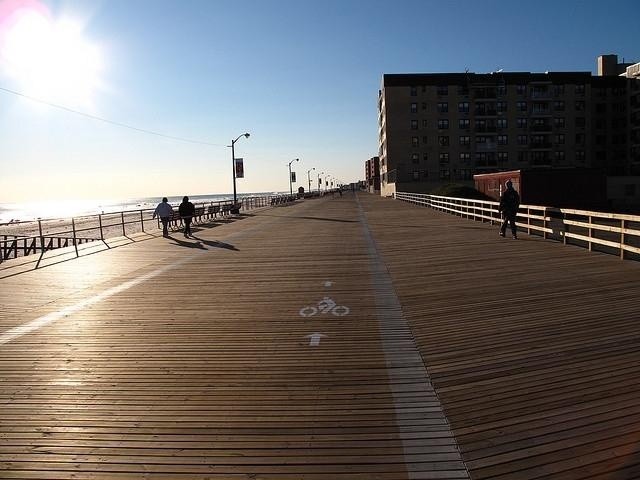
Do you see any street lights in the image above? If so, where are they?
[317,171,338,191]
[308,168,315,193]
[285,158,299,196]
[227,133,250,205]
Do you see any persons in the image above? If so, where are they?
[497,179,520,240]
[152,196,195,238]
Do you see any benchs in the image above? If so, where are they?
[155,202,242,233]
[268,194,298,205]
